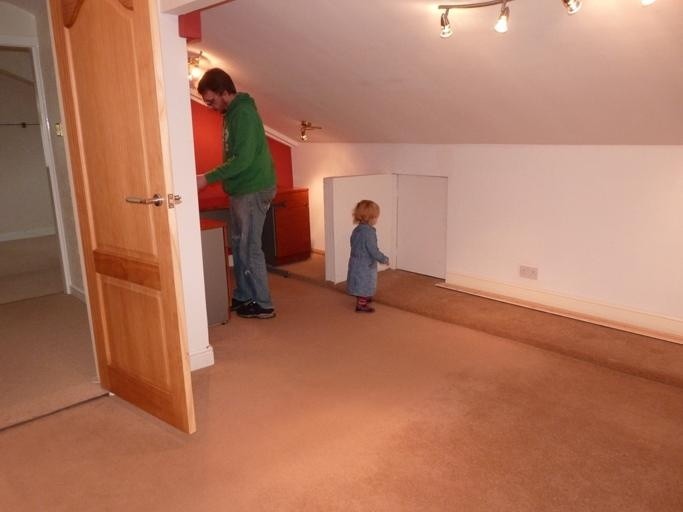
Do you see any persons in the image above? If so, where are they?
[195,67,278,318]
[345,199,390,314]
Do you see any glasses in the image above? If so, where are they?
[205,97,215,106]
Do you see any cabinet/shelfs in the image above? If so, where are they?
[190,185,311,328]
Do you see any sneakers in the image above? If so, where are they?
[230,297,274,318]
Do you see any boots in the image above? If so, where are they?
[356,296,375,312]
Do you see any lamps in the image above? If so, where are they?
[187,51,204,81]
[299,120,322,141]
[438,0,583,38]
